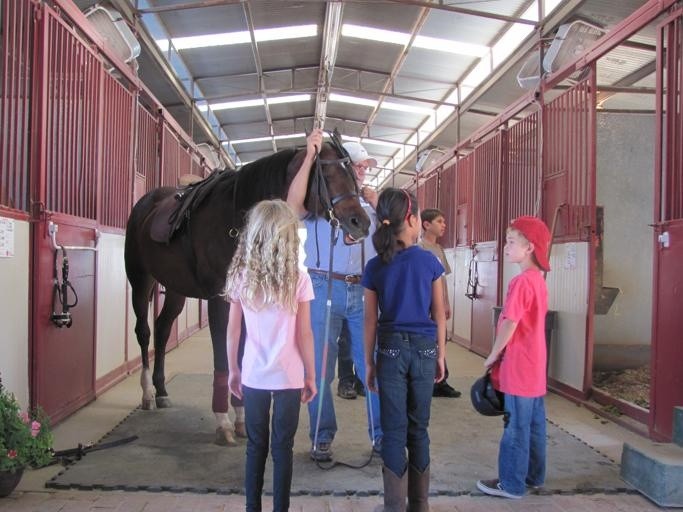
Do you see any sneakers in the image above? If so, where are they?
[431,381,464,401]
[335,378,360,400]
[306,441,336,463]
[476,475,525,502]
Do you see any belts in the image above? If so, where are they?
[311,268,363,286]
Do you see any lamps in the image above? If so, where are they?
[415,149,446,173]
[72,3,141,74]
[516,19,607,89]
[186,142,227,173]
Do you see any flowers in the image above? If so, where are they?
[0,373,56,474]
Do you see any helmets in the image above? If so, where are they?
[468,370,514,430]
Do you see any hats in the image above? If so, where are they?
[339,141,378,172]
[508,213,554,274]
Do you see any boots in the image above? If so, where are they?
[373,461,433,511]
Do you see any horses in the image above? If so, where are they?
[123,126,371,447]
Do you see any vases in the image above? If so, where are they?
[0,464,26,498]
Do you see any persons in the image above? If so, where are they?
[417,207,462,397]
[477,214,552,499]
[337,320,366,399]
[288,127,384,462]
[218,197,317,512]
[362,186,447,512]
